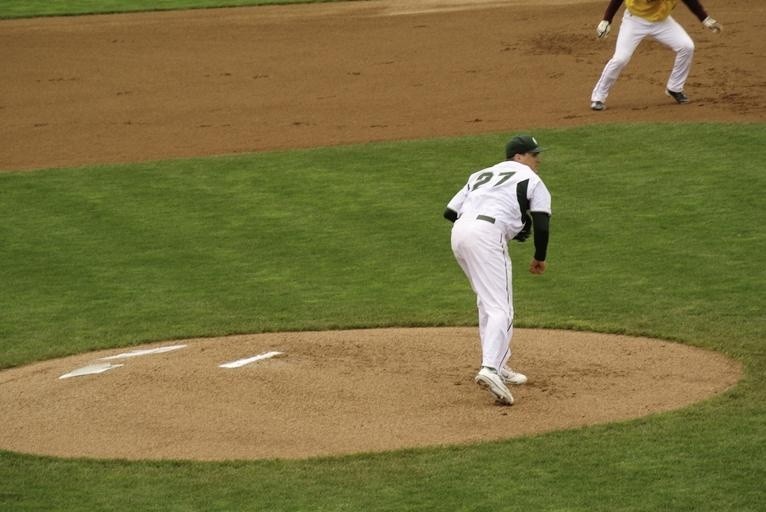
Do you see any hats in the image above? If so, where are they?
[506,136,549,160]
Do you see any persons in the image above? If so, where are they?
[442,135,552,406]
[589,0,723,112]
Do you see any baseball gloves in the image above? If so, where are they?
[514,214,532,242]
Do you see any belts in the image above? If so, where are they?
[457,212,494,224]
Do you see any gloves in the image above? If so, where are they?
[597,19,612,40]
[701,16,723,37]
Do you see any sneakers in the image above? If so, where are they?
[665,88,688,103]
[590,101,604,110]
[476,365,528,405]
